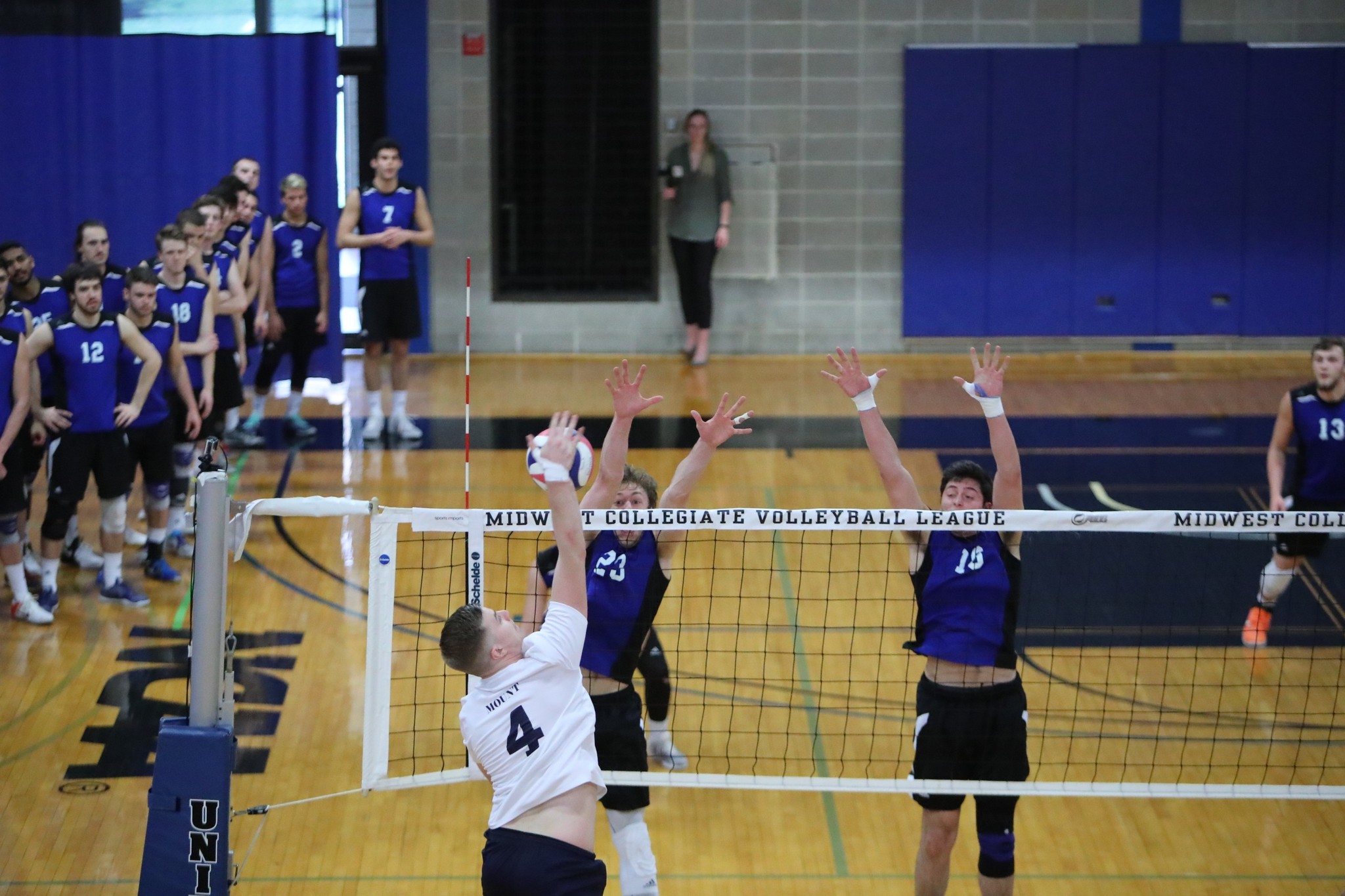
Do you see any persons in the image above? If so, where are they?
[821,343,1031,896]
[333,136,435,440]
[660,109,734,364]
[1241,333,1345,647]
[440,359,756,896]
[0,158,330,625]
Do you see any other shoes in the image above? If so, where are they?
[679,344,697,354]
[225,424,265,449]
[649,738,688,768]
[241,414,263,432]
[39,587,59,611]
[101,577,150,606]
[285,416,318,436]
[123,526,147,546]
[387,412,423,440]
[167,530,194,558]
[362,415,385,440]
[5,562,42,591]
[143,558,182,582]
[73,548,104,569]
[691,352,709,366]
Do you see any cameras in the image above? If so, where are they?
[660,165,683,188]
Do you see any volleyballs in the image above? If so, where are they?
[525,427,595,494]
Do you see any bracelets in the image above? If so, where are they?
[720,223,732,231]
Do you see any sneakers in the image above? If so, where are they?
[1242,606,1272,647]
[10,594,54,624]
